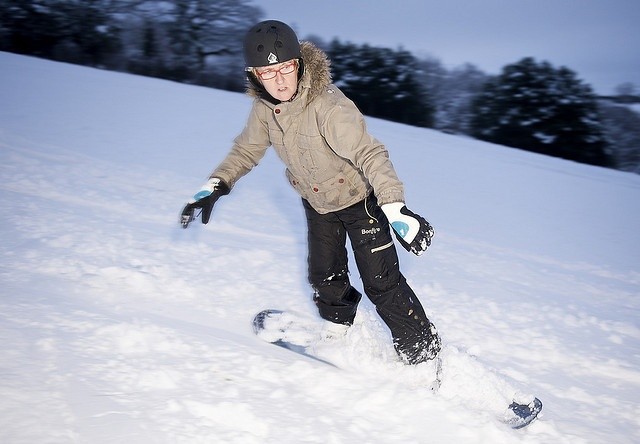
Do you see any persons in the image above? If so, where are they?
[179,20,445,392]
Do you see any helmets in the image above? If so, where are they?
[242,20,304,67]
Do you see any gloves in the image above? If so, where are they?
[180,178,231,230]
[383,201,434,256]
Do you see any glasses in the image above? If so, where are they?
[254,60,297,81]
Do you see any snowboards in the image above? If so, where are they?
[252,309,542,429]
